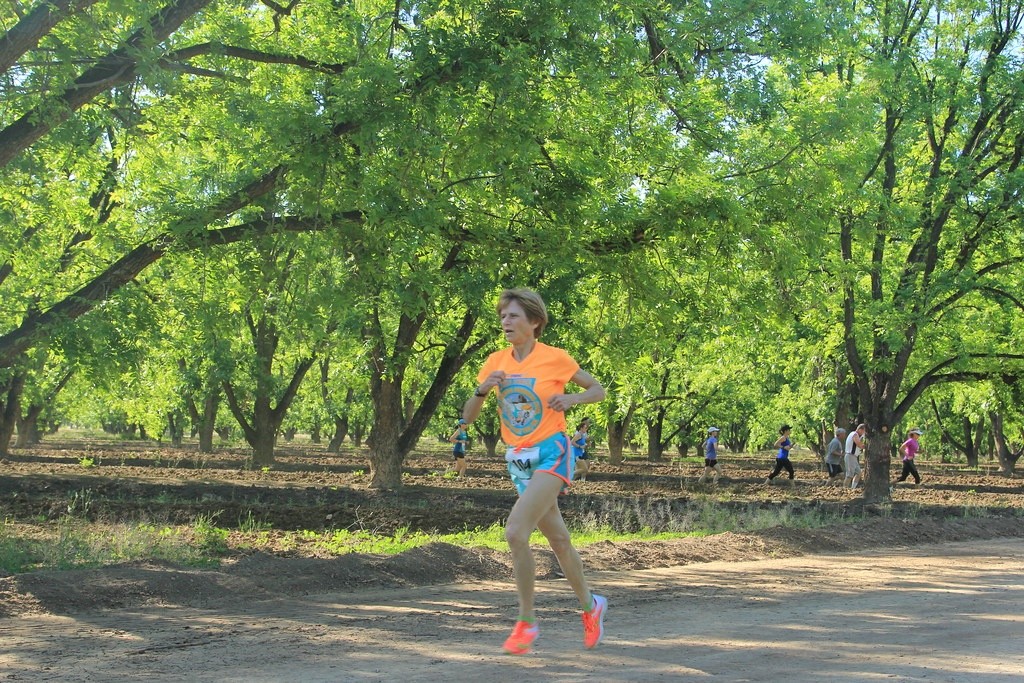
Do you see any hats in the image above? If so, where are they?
[781,425,792,431]
[458,419,465,425]
[709,427,720,433]
[910,430,923,435]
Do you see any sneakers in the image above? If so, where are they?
[502,620,542,655]
[581,594,608,651]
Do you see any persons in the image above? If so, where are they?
[764,425,794,487]
[571,417,592,481]
[825,428,847,487]
[844,424,866,489]
[890,429,925,492]
[463,290,608,656]
[440,419,469,482]
[699,427,722,485]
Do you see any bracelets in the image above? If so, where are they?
[579,445,580,448]
[475,388,488,397]
